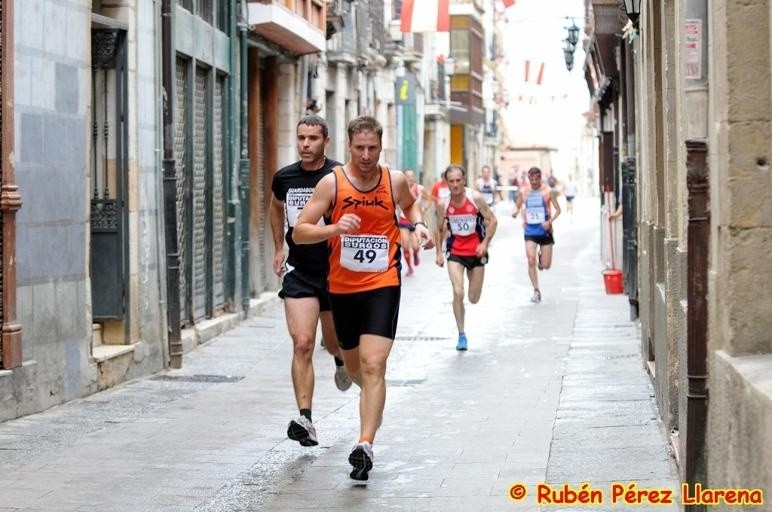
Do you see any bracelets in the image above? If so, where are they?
[423,206,428,211]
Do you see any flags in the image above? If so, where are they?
[493,1,515,12]
[523,60,545,86]
[399,0,452,34]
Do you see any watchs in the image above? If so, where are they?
[413,220,429,229]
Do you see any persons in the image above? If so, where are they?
[431,165,578,253]
[392,167,433,277]
[268,113,355,448]
[607,200,623,222]
[434,163,498,351]
[290,113,437,481]
[511,167,561,303]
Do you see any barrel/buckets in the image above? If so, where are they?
[600,268,624,294]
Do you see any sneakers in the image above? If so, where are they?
[456,334,467,350]
[287,416,318,446]
[531,288,541,302]
[348,441,373,480]
[334,357,351,391]
[538,252,543,270]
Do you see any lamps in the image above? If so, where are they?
[561,14,591,71]
[623,0,641,24]
